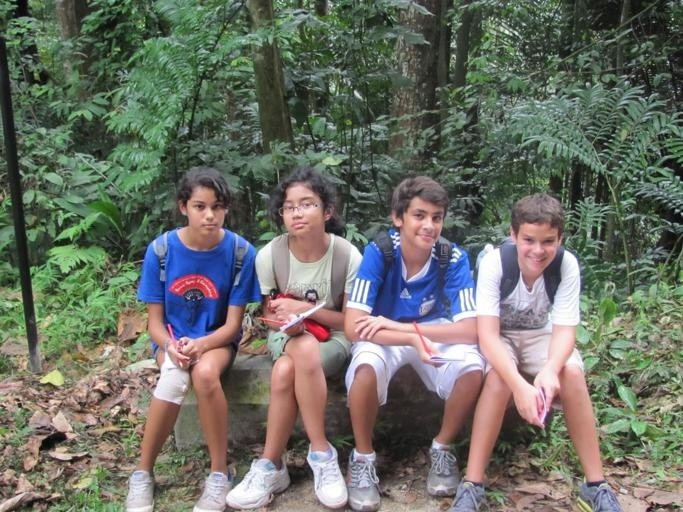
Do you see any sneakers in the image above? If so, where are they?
[225,459,290,510]
[192,472,233,512]
[576,476,624,512]
[126,471,155,512]
[446,476,484,512]
[306,442,347,509]
[426,447,459,497]
[345,449,380,512]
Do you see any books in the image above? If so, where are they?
[429,346,467,366]
[533,385,552,423]
[169,359,202,367]
[278,299,329,333]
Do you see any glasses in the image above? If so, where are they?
[278,200,324,216]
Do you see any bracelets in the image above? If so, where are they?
[164,340,173,361]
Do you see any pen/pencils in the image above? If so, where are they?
[256,316,289,325]
[412,321,433,357]
[167,323,184,368]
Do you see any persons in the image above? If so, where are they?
[450,190,625,510]
[118,165,256,511]
[225,169,363,510]
[349,175,483,509]
